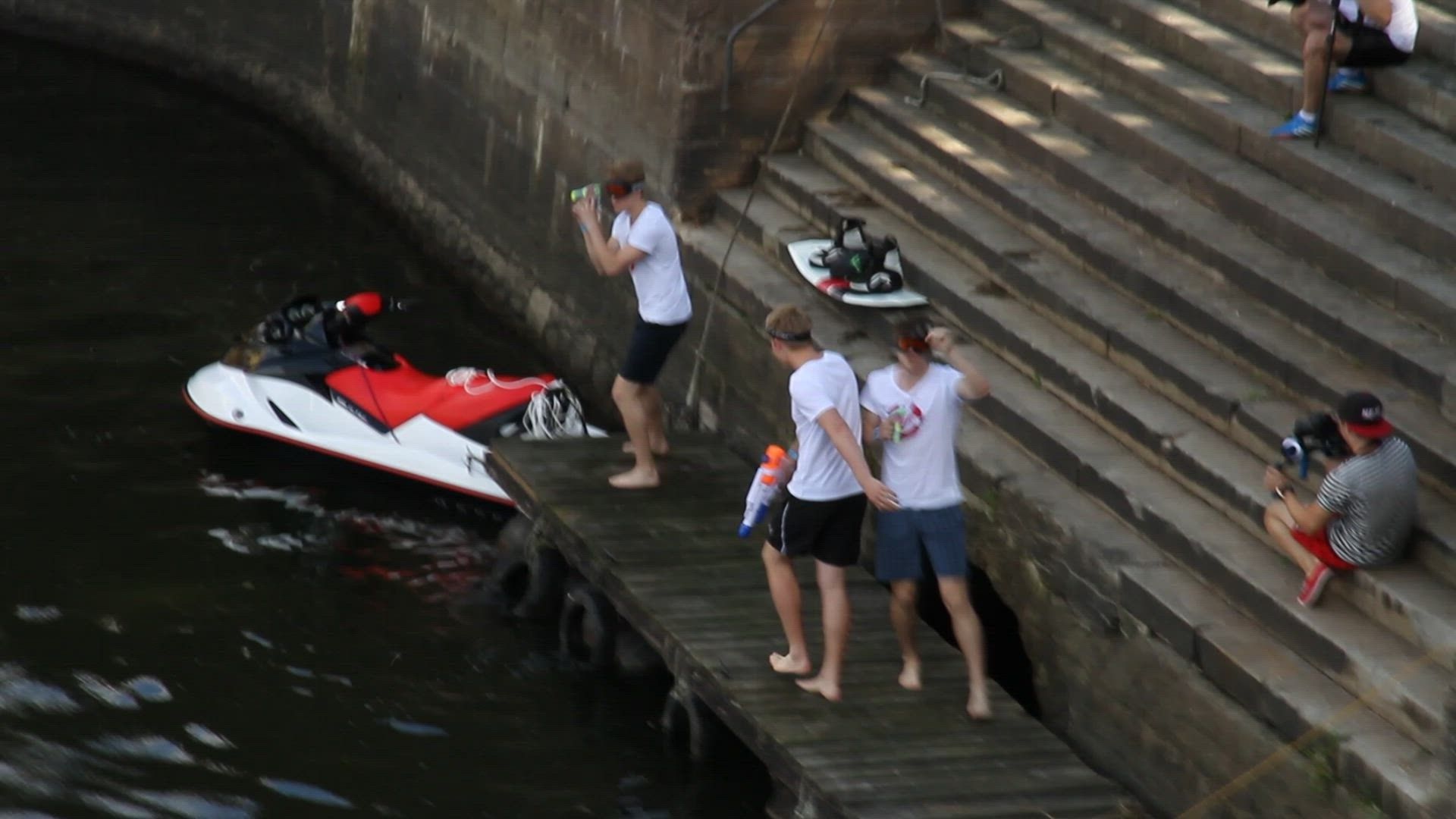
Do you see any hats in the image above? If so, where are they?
[1339,391,1391,437]
[1294,411,1352,460]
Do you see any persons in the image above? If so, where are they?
[1264,392,1422,606]
[858,310,991,721]
[1268,0,1420,140]
[760,309,901,702]
[570,160,694,491]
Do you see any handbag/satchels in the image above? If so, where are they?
[829,216,904,292]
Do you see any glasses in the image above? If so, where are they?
[606,179,633,196]
[895,335,930,356]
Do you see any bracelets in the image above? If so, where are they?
[873,425,882,442]
[580,223,587,232]
[1273,485,1296,500]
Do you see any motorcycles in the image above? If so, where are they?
[180,289,613,526]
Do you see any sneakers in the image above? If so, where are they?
[1299,562,1333,610]
[1269,110,1319,140]
[1327,71,1367,91]
[809,246,846,267]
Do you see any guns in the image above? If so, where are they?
[735,443,789,540]
